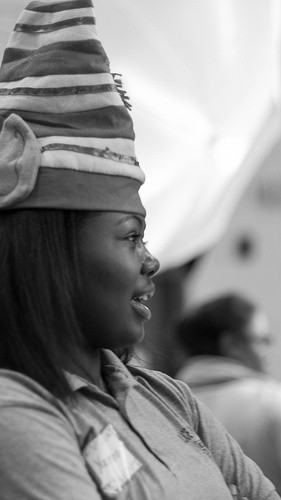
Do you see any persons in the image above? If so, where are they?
[172,294,281,500]
[0,85,281,499]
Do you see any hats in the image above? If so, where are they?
[0,0,146,215]
